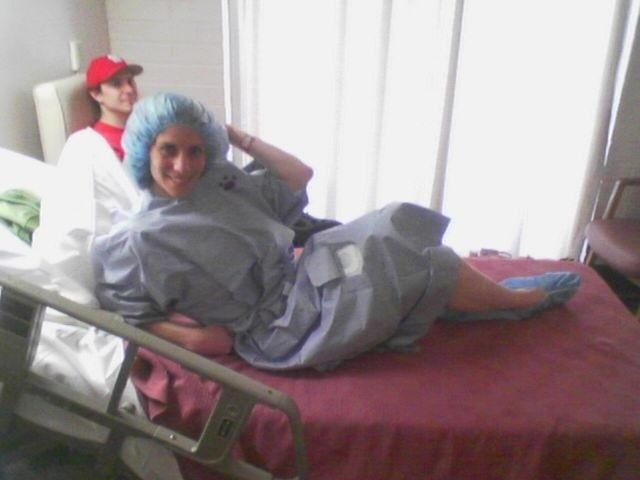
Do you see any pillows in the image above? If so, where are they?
[31,156,139,328]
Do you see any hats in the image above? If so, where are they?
[85,51,146,91]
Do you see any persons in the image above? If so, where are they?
[85,55,342,246]
[89,91,582,375]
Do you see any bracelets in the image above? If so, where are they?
[240,134,251,153]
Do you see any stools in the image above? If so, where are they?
[576,175,640,292]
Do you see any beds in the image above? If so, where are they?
[0,145,640,480]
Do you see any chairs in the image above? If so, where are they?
[31,72,104,167]
